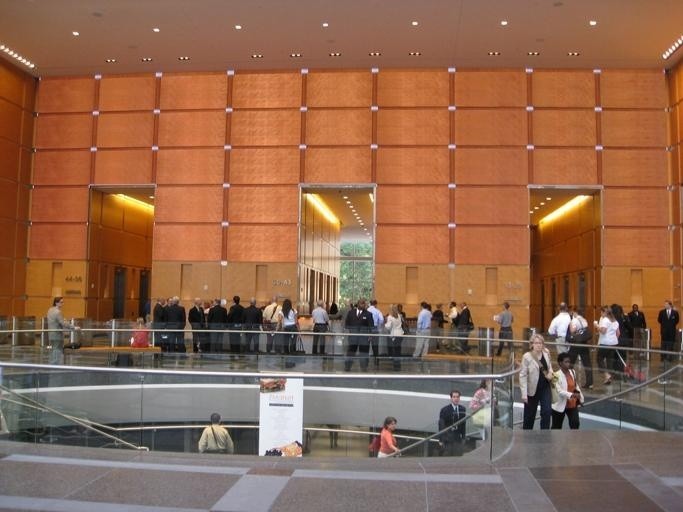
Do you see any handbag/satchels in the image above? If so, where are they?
[263,320,271,331]
[468,322,474,330]
[573,327,593,343]
[549,373,558,403]
[368,436,381,452]
[401,321,410,334]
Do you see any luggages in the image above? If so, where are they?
[616,351,646,381]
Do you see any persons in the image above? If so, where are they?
[378,417,400,456]
[657,299,679,362]
[47,297,80,364]
[344,299,408,372]
[438,391,467,456]
[519,333,554,431]
[312,300,331,354]
[413,300,472,357]
[131,297,298,354]
[548,302,646,389]
[471,378,498,441]
[550,353,584,430]
[494,302,514,356]
[327,424,340,449]
[197,413,234,454]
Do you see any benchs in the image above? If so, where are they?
[423,353,507,374]
[80,346,161,369]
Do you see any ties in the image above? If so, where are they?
[454,407,458,412]
